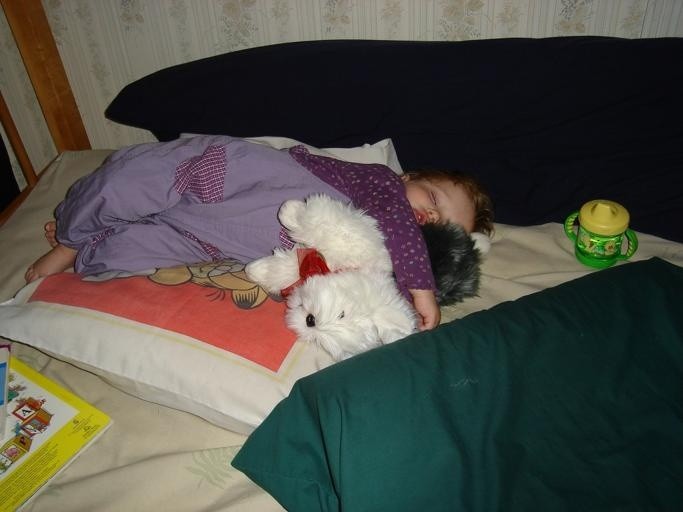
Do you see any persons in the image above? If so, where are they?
[25,136,493,332]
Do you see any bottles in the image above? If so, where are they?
[564,200,638,268]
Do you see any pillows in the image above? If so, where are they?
[0,137,405,437]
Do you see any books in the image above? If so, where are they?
[0,345,113,512]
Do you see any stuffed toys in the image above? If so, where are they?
[245,194,480,359]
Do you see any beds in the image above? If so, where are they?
[0,150,683,512]
